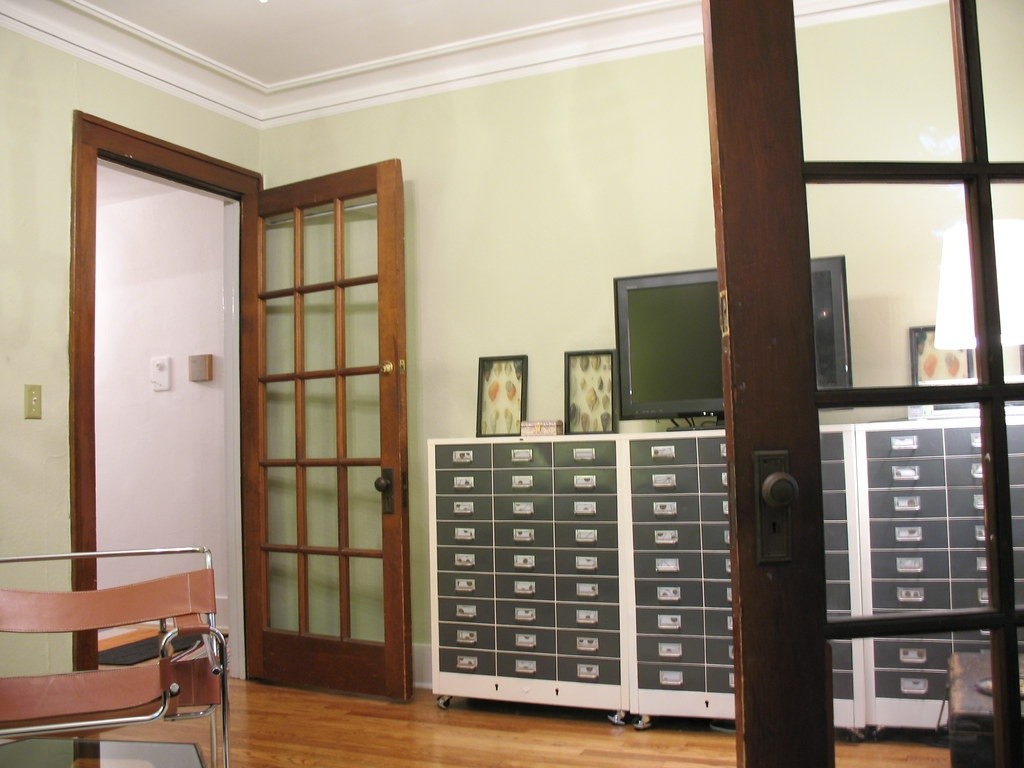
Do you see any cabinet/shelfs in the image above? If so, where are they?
[427,434,630,726]
[624,422,867,743]
[854,415,1024,744]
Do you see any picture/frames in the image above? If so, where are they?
[476,354,528,438]
[564,351,618,435]
[908,325,974,411]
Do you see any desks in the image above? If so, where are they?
[946,651,1024,768]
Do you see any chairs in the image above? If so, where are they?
[0,544,231,768]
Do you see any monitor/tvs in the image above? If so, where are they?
[613,254,851,431]
